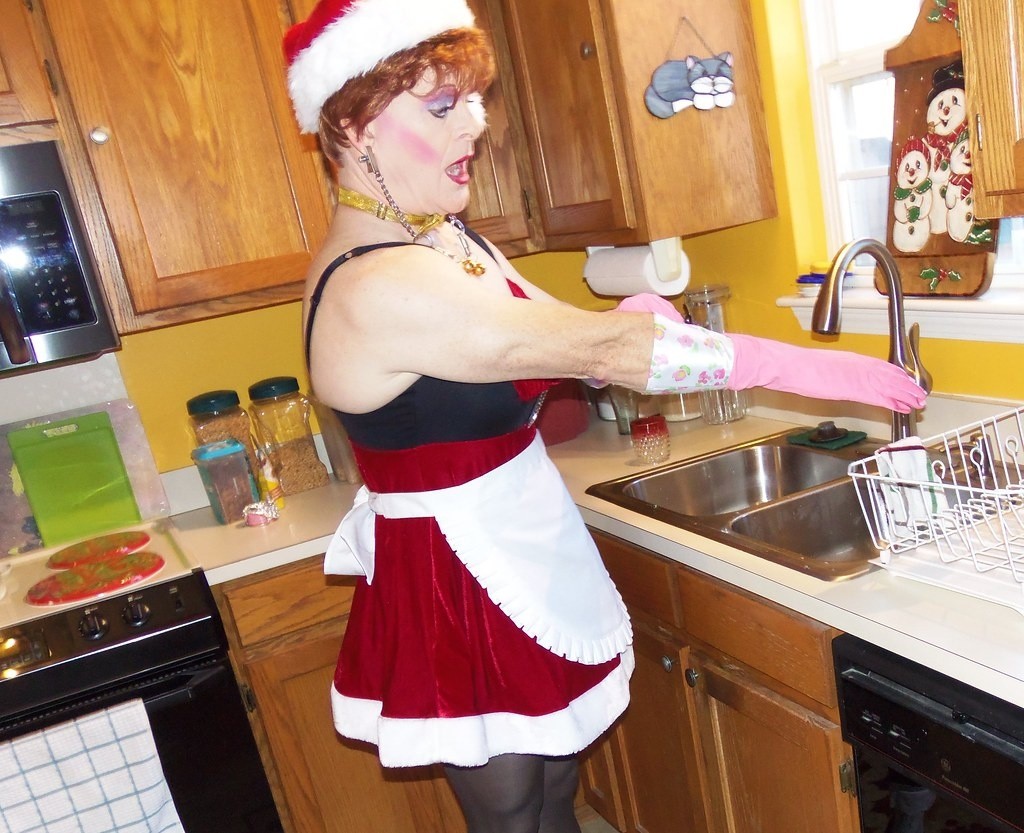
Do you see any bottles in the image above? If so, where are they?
[603,384,639,435]
[682,281,749,426]
[187,375,331,493]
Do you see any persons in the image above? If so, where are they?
[282,0,929,833]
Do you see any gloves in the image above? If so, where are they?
[580,293,686,390]
[641,311,927,413]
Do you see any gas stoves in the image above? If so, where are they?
[0,521,235,683]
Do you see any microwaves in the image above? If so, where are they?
[0,140,121,369]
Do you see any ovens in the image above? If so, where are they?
[0,646,272,833]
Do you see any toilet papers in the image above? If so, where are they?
[582,245,691,297]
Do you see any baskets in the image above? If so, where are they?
[847,408,1021,582]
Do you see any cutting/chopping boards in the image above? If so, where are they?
[7,411,140,550]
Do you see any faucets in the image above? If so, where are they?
[810,238,934,443]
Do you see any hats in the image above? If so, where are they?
[282,0,474,135]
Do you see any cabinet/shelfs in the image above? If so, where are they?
[211,518,862,833]
[0,1,778,336]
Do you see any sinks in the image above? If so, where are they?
[730,473,1015,563]
[622,444,864,518]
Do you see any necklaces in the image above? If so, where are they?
[338,185,445,236]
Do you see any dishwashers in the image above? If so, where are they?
[834,634,1024,833]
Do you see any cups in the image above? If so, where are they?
[629,415,670,462]
